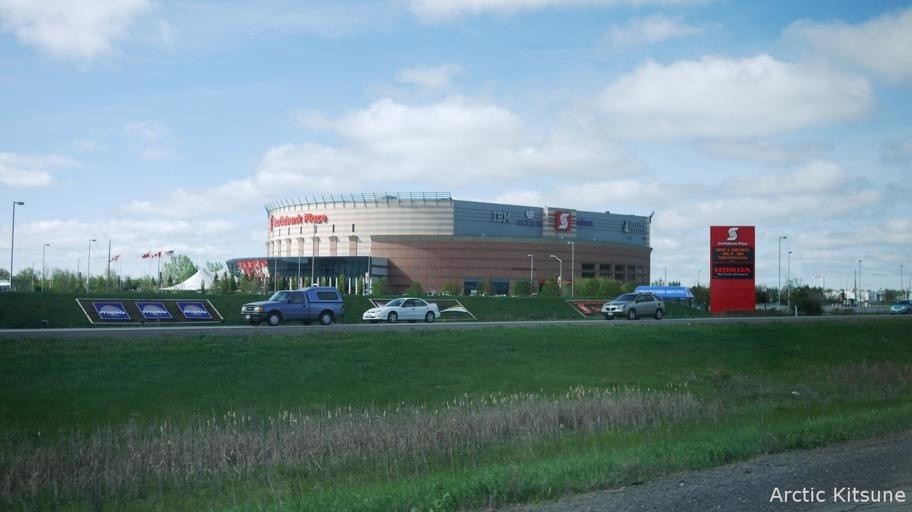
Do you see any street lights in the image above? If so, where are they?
[87,239,96,294]
[858,259,863,307]
[778,236,786,307]
[786,251,792,308]
[158,250,174,287]
[9,201,24,290]
[550,254,563,289]
[527,254,534,286]
[568,240,575,298]
[42,244,50,292]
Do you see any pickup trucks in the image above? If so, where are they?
[241,287,344,325]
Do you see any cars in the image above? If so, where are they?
[888,299,912,313]
[601,292,666,319]
[362,297,441,323]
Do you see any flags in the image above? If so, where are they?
[164,250,175,256]
[142,251,150,259]
[152,251,160,259]
[109,254,120,261]
